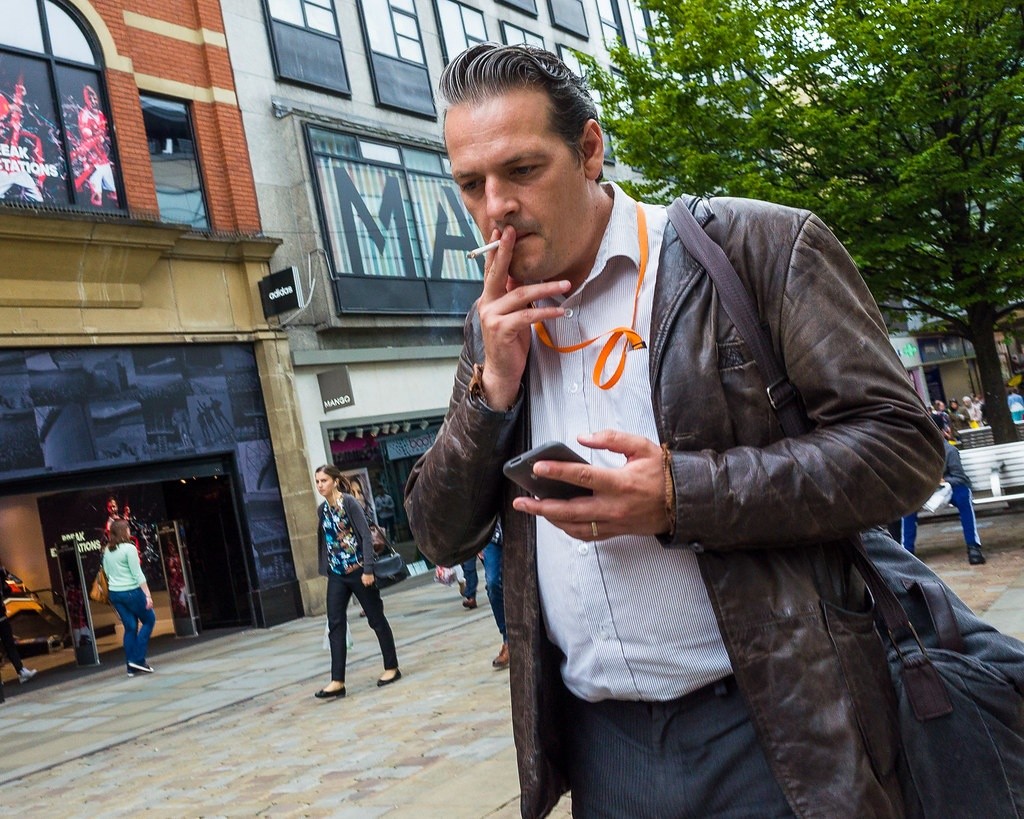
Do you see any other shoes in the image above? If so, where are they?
[492,642,510,667]
[461,597,476,608]
[965,544,985,564]
[314,686,346,697]
[377,668,402,687]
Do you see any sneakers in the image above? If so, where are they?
[126,671,138,676]
[19,668,37,684]
[128,659,153,673]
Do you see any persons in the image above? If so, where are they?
[375,485,395,545]
[0,557,37,683]
[102,519,156,676]
[71,85,119,208]
[105,497,143,567]
[888,389,1024,564]
[404,44,945,819]
[347,474,375,528]
[0,105,45,202]
[455,516,509,667]
[315,466,401,698]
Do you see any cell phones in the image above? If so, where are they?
[501,442,593,499]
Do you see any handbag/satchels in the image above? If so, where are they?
[89,568,110,605]
[373,553,410,590]
[434,564,457,587]
[855,523,1024,819]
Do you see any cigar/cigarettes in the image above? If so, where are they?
[466,240,500,258]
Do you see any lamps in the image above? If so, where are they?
[337,428,348,442]
[328,429,335,441]
[419,419,429,430]
[355,426,364,439]
[390,422,400,434]
[382,422,390,435]
[402,420,411,433]
[370,424,379,437]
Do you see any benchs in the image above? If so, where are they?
[916,419,1024,518]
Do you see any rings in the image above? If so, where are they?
[591,521,598,537]
